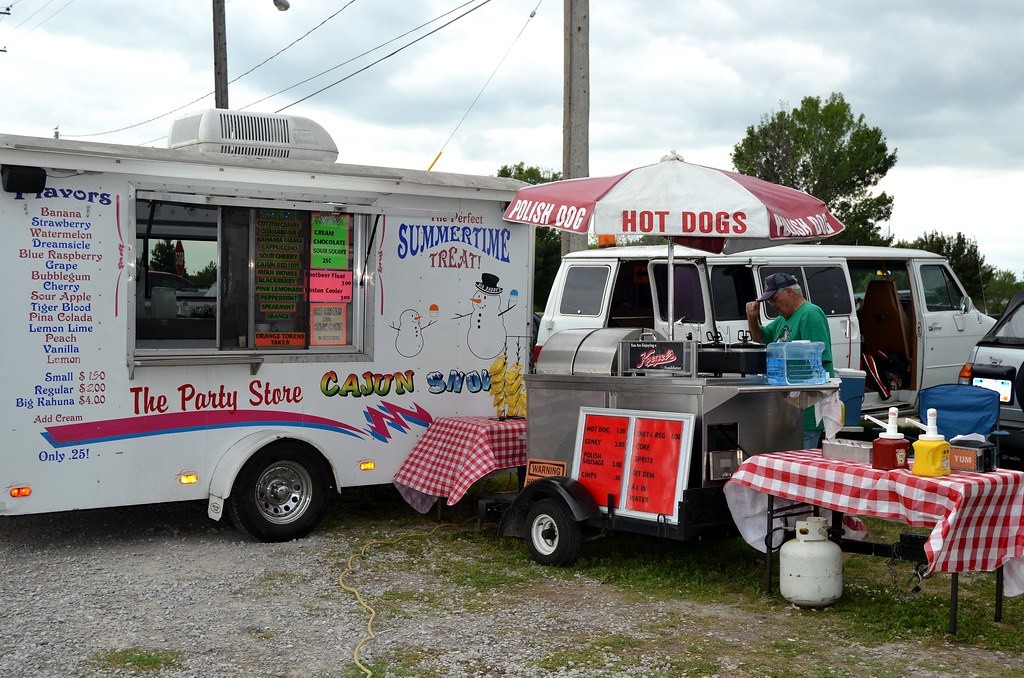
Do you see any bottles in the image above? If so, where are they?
[905,408,951,477]
[766,339,826,387]
[864,406,909,471]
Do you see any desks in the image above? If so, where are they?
[435,415,527,532]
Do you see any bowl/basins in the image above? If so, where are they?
[255,323,270,332]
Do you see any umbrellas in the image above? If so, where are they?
[502,151,846,342]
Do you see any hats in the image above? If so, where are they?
[756,273,797,302]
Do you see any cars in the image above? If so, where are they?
[957,291,1023,470]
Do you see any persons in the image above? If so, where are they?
[746,272,835,449]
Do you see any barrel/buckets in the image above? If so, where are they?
[149,287,177,319]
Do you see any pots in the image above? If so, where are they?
[698,331,729,373]
[726,330,766,372]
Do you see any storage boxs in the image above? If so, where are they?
[947,439,997,474]
[833,368,867,427]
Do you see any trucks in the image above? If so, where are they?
[1,109,536,541]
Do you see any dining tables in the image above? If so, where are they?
[750,448,1024,637]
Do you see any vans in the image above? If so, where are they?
[534,244,999,431]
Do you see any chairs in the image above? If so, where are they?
[918,383,1001,468]
[862,280,912,372]
